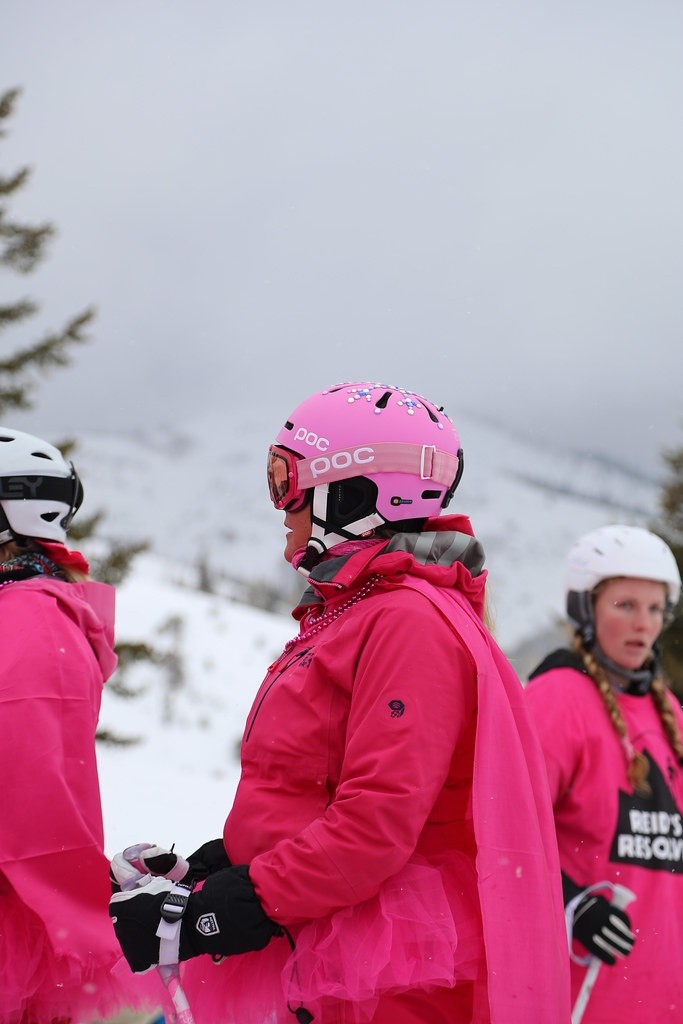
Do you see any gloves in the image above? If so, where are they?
[110,838,233,894]
[109,863,285,975]
[559,869,635,967]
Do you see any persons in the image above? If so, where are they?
[110,380,571,1024]
[0,427,145,1024]
[524,526,683,1024]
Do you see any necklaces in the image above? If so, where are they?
[285,574,382,647]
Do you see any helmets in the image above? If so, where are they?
[0,426,84,546]
[275,382,464,524]
[564,526,681,628]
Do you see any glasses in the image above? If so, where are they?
[266,445,307,514]
